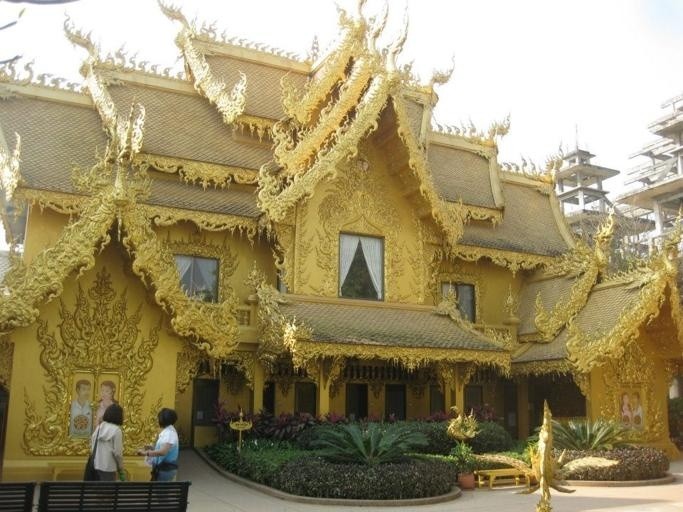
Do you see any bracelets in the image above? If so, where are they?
[147,451,149,456]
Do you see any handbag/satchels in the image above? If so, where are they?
[84,453,97,480]
[150,453,159,481]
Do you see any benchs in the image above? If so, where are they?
[0,481,36,512]
[39,482,191,512]
[476,468,527,489]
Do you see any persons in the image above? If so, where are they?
[91,404,126,482]
[96,381,119,428]
[137,407,179,504]
[69,380,91,436]
[631,392,642,425]
[620,392,631,424]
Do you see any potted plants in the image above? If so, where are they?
[451,440,476,490]
